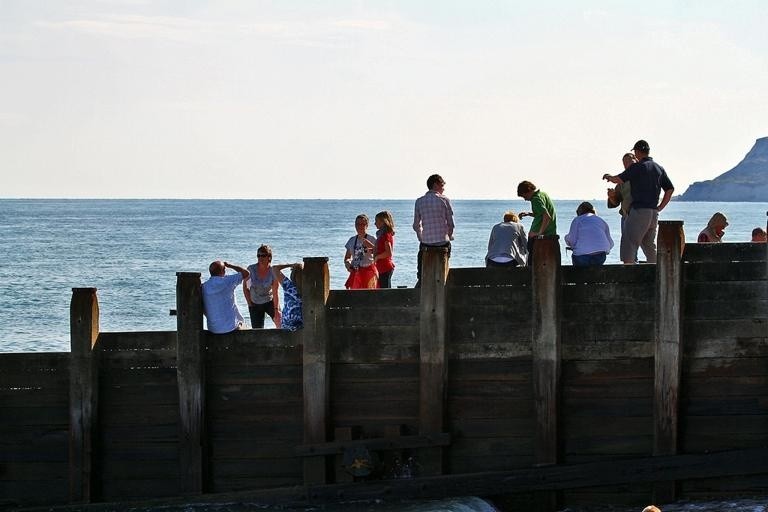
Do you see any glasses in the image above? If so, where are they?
[257,254,268,257]
[356,223,367,227]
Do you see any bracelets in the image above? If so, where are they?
[274,307,279,310]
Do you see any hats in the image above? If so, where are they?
[577,202,596,216]
[631,140,650,151]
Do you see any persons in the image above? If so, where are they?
[344,215,377,290]
[606,153,639,263]
[517,180,557,265]
[698,213,727,242]
[485,212,527,269]
[201,260,251,334]
[243,244,282,329]
[412,174,455,288]
[272,263,303,332]
[603,140,674,264]
[565,203,614,267]
[363,211,395,288]
[750,228,766,241]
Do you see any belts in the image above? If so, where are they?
[421,241,450,247]
